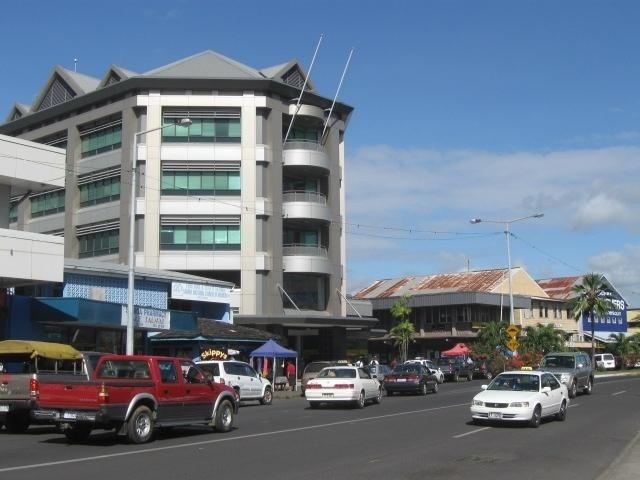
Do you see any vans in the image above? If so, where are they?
[538,351,594,399]
[595,353,616,369]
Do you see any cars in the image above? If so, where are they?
[185,359,274,405]
[473,359,496,380]
[301,359,445,409]
[470,366,570,427]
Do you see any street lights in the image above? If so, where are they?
[469,213,544,357]
[126,118,193,355]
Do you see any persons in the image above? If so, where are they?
[467,356,473,364]
[356,359,363,367]
[370,357,379,365]
[286,361,296,391]
[391,358,398,369]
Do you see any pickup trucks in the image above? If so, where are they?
[435,357,474,382]
[0,339,240,444]
